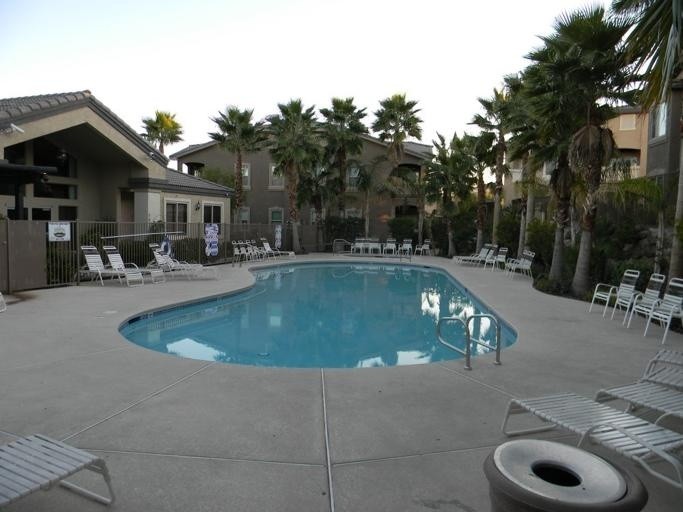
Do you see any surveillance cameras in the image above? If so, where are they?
[10,123,24,134]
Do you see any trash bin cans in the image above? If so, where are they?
[484,436,648,512]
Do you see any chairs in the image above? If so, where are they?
[589,269,683,344]
[629,365,683,412]
[77,242,224,287]
[452,243,536,281]
[0,432,116,509]
[231,237,297,268]
[643,349,683,376]
[350,238,433,257]
[501,391,683,491]
[350,263,430,282]
[588,381,683,446]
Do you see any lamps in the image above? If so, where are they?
[195,200,200,211]
